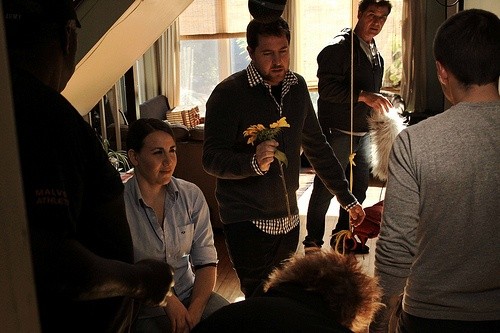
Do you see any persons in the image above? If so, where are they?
[1,0,177,333]
[120,118,232,333]
[188,249,383,333]
[368,8,500,333]
[303,0,393,254]
[201,15,366,300]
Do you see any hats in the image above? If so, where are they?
[2,0,81,52]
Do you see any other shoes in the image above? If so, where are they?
[330,230,369,254]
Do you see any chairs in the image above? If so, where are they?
[140,95,171,122]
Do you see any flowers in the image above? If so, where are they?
[242,116,292,225]
